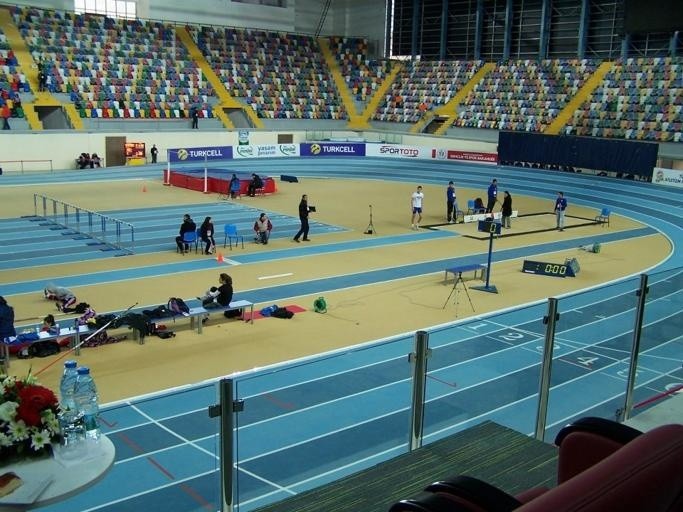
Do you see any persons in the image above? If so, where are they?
[554,192,567,231]
[447,178,512,229]
[79,152,102,169]
[197,273,233,307]
[246,173,263,197]
[176,214,196,253]
[44,314,54,328]
[151,144,157,163]
[0,296,16,359]
[294,195,311,242]
[411,186,424,229]
[228,173,240,198]
[192,107,199,129]
[200,217,215,254]
[254,213,272,244]
[38,69,47,92]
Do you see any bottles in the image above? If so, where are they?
[60,360,100,438]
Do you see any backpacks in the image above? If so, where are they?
[168,297,190,314]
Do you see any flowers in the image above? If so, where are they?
[0,366,84,490]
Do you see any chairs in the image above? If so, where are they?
[177,228,216,254]
[256,178,266,197]
[467,199,486,215]
[226,179,241,199]
[223,224,245,250]
[389,414,682,512]
[594,207,610,227]
[75,157,105,169]
[0,0,682,141]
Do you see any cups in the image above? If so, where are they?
[62,425,87,459]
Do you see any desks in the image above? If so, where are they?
[1,427,116,508]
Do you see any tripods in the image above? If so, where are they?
[363,205,377,235]
[217,175,226,200]
[442,271,475,319]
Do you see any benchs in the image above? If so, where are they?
[444,263,487,285]
[7,299,253,366]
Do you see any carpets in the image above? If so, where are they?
[282,418,559,496]
